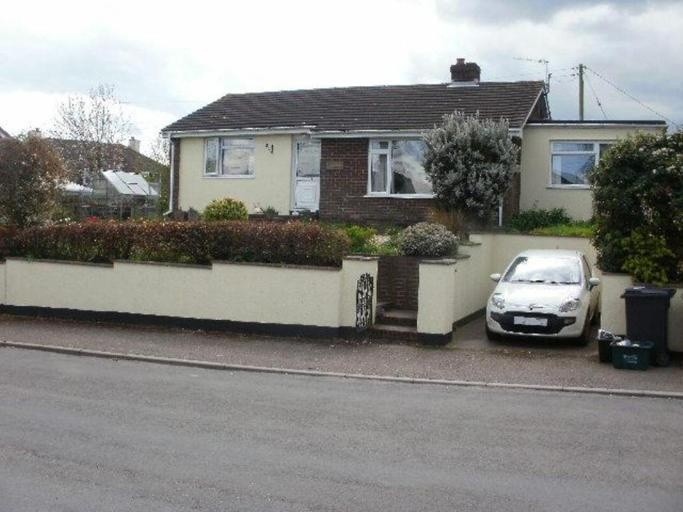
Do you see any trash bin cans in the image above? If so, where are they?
[620,286,676,366]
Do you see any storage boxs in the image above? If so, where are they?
[599,334,624,362]
[611,341,655,369]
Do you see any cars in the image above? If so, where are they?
[484,247,601,347]
